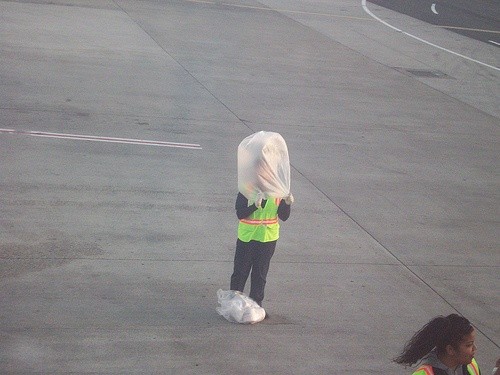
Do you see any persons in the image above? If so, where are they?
[389,313,483,375]
[230,130,294,319]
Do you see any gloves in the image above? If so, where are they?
[255,194,263,208]
[285,193,293,205]
